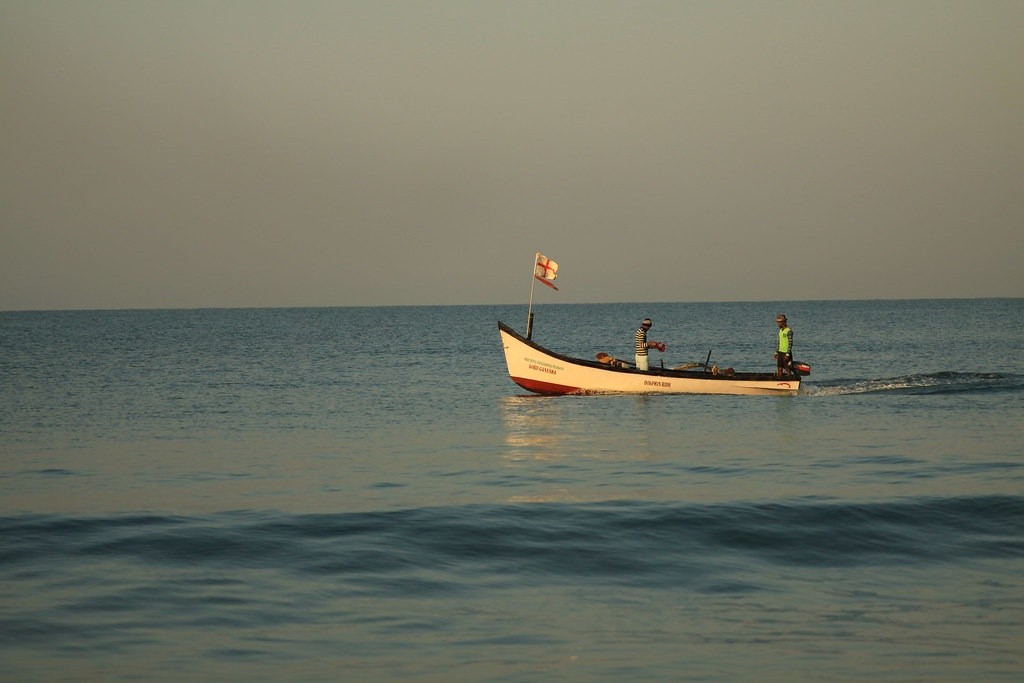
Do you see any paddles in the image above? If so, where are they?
[596,352,637,366]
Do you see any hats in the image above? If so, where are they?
[777,314,788,322]
[643,319,652,327]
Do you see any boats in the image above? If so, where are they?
[497,252,803,396]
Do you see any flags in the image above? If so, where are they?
[535,252,558,281]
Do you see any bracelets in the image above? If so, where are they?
[786,354,790,357]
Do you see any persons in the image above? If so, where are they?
[635,318,657,372]
[774,315,793,380]
